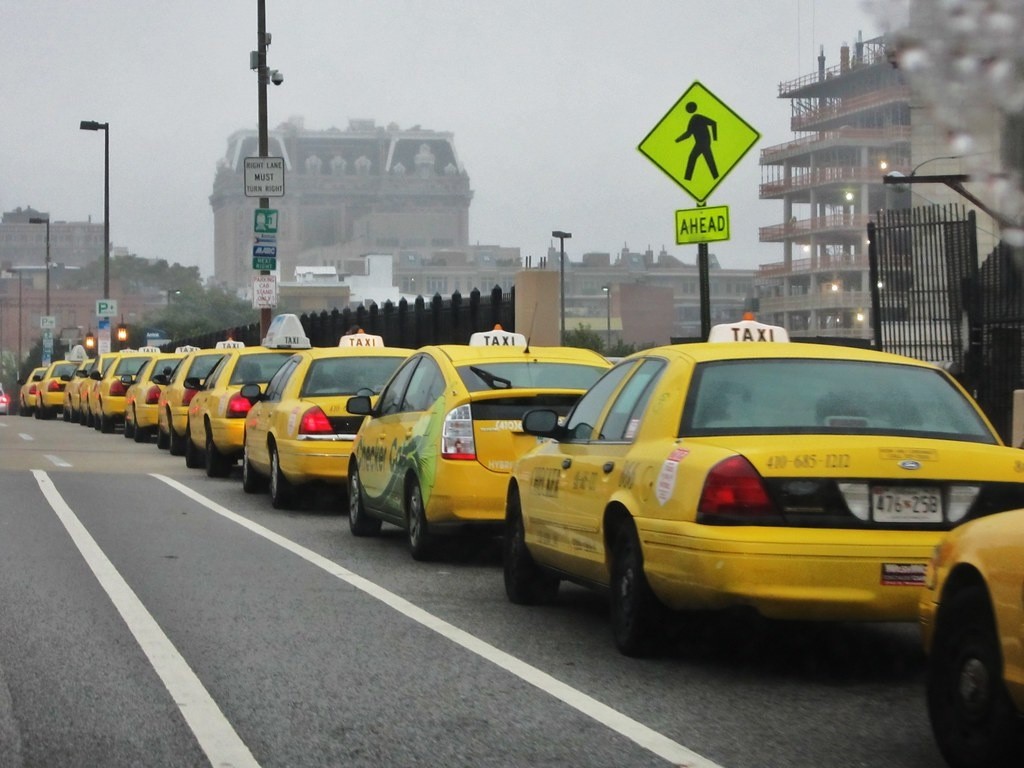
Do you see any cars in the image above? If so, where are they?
[183,313,322,478]
[76,347,138,428]
[153,337,246,455]
[918,508,1024,768]
[17,367,49,417]
[240,328,418,510]
[90,345,161,434]
[346,324,614,563]
[0,383,8,415]
[60,358,95,423]
[121,345,201,443]
[33,345,89,420]
[503,312,1024,656]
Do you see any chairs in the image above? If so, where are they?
[241,363,262,382]
[697,380,758,424]
[817,390,869,424]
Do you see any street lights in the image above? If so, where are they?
[7,269,21,365]
[85,325,95,359]
[552,231,571,346]
[80,121,110,321]
[117,314,127,349]
[30,218,50,333]
